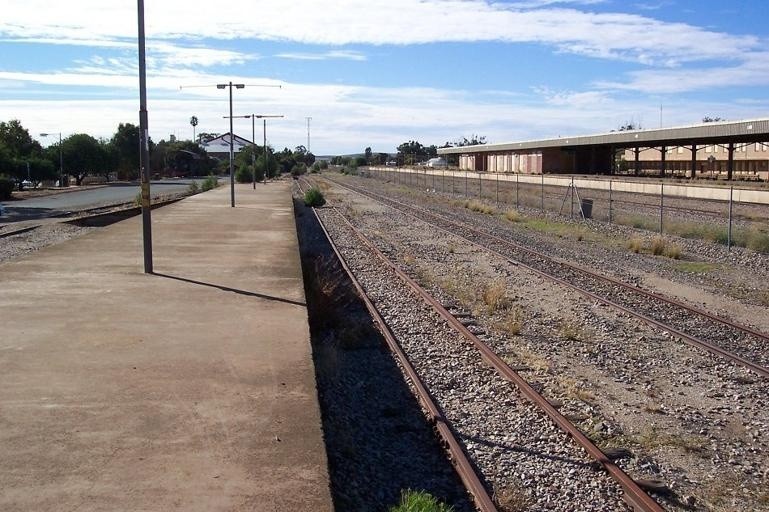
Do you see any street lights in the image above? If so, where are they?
[244,113,262,190]
[217,82,246,209]
[304,116,314,153]
[40,131,64,186]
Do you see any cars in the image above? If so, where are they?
[11,178,38,192]
